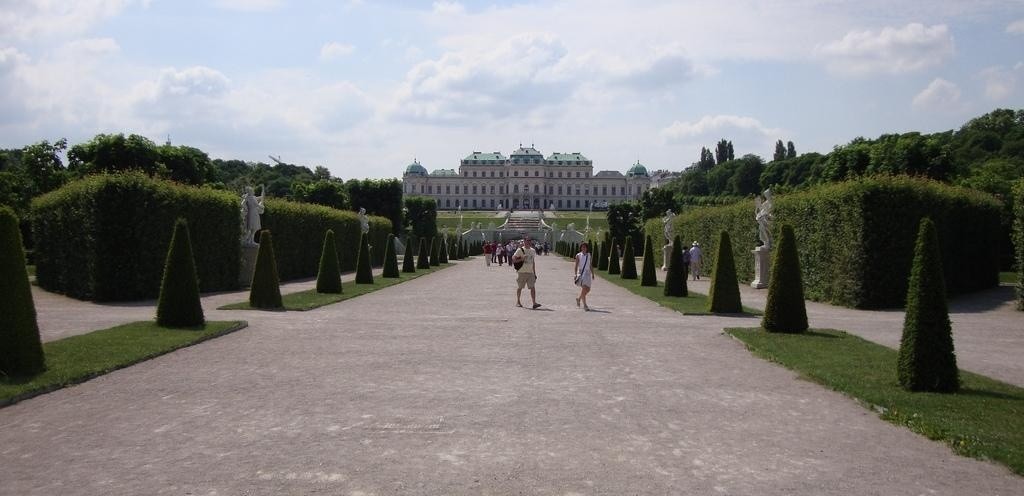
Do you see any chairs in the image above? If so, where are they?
[516,298,591,312]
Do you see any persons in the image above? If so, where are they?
[682,246,691,279]
[482,238,549,267]
[512,236,542,309]
[574,243,595,311]
[663,209,675,245]
[241,186,264,247]
[755,188,772,248]
[689,241,701,281]
[359,208,369,232]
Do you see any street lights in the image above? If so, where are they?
[512,247,526,271]
[574,273,582,287]
